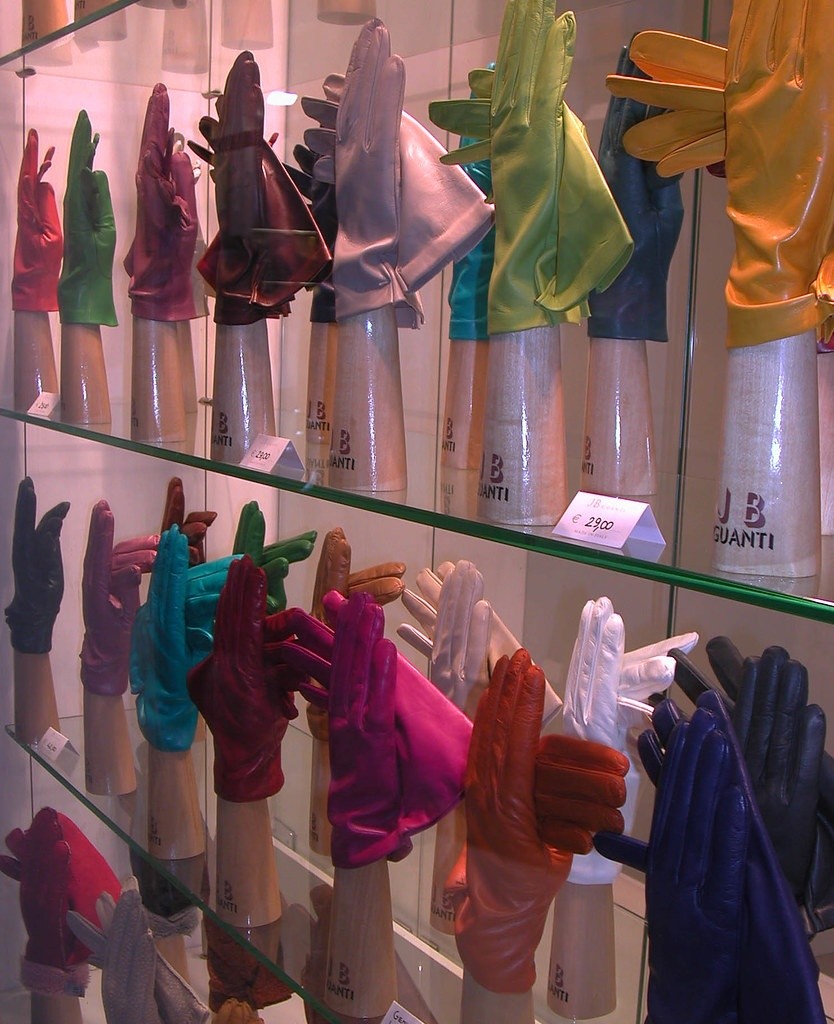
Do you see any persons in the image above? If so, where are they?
[5,0,833,1023]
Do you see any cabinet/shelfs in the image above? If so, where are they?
[2,2,834,1024]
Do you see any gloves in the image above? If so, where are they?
[1,477,834,1024]
[10,0,834,351]
